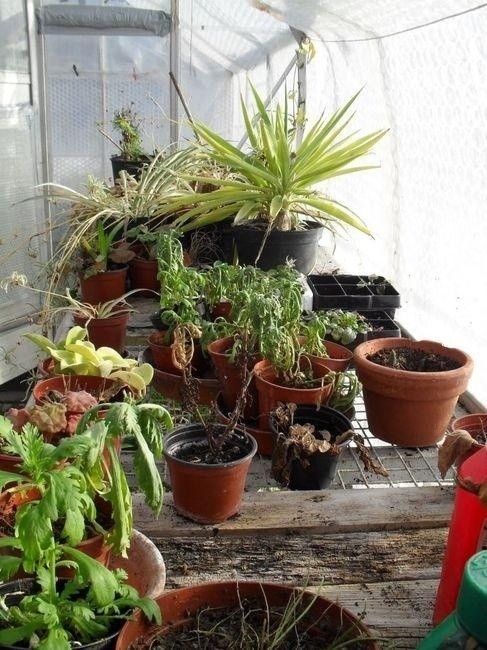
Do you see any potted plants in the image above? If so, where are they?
[4,67,387,308]
[156,232,377,492]
[2,324,155,476]
[2,412,115,566]
[353,337,472,446]
[2,270,165,356]
[1,402,178,649]
[165,320,264,525]
[115,573,391,648]
